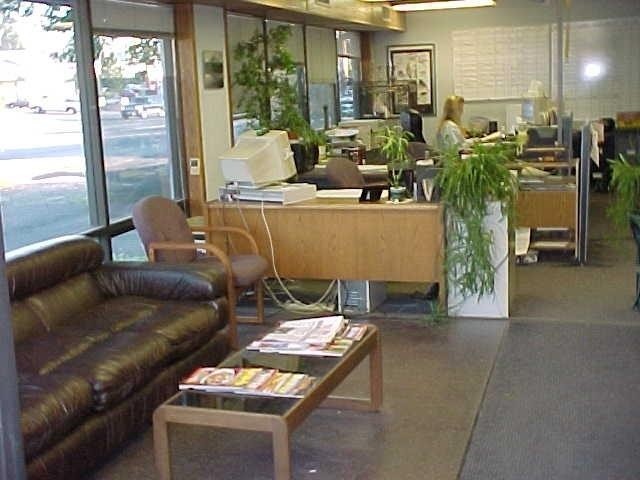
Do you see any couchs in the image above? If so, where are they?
[2,236,232,478]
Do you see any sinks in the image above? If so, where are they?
[2,236,232,478]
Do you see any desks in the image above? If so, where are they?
[153,322,383,478]
[213,119,582,316]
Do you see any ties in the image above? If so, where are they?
[218,130,298,189]
[521,95,544,124]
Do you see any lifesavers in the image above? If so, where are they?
[337,279,390,315]
[506,104,521,130]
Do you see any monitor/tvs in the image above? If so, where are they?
[517,173,577,191]
[244,315,369,359]
[173,365,321,401]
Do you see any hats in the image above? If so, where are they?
[6,82,165,119]
[340,96,355,115]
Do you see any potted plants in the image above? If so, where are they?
[387,44,436,115]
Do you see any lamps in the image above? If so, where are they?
[131,195,267,324]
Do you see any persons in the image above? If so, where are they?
[436,94,504,154]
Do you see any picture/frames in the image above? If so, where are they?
[153,322,383,478]
[387,44,436,115]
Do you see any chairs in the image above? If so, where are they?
[131,195,267,324]
